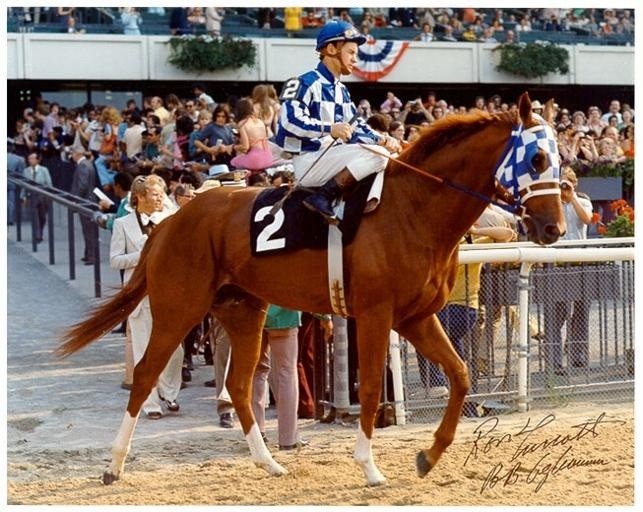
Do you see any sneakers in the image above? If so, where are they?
[408,387,447,398]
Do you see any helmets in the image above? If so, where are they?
[316,19,366,51]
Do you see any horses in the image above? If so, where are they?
[47,92,567,486]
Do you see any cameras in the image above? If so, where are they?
[409,100,418,107]
[559,178,568,191]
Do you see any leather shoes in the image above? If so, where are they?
[204,378,215,387]
[220,412,233,428]
[120,367,191,418]
[279,438,309,451]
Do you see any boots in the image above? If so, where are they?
[301,176,344,226]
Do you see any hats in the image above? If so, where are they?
[531,100,541,109]
[69,145,93,157]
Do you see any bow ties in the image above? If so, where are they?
[140,212,161,227]
